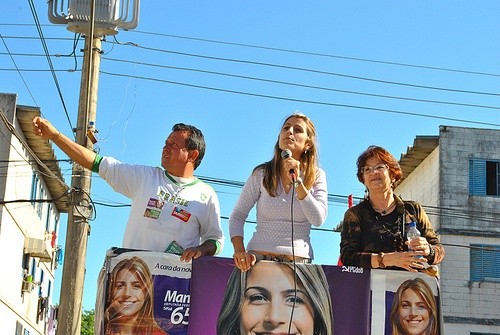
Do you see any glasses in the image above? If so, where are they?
[361,164,389,173]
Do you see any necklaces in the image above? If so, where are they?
[376,214,403,252]
[370,198,396,213]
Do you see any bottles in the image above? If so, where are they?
[407,222,424,259]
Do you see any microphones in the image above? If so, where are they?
[281,149,295,179]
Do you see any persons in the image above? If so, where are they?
[228,112,327,272]
[33,116,224,263]
[215,258,333,335]
[343,145,446,273]
[391,277,438,335]
[107,256,168,335]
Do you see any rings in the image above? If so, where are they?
[241,258,245,262]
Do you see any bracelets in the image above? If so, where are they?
[377,252,385,269]
[291,178,303,187]
[52,132,63,142]
[423,243,434,259]
